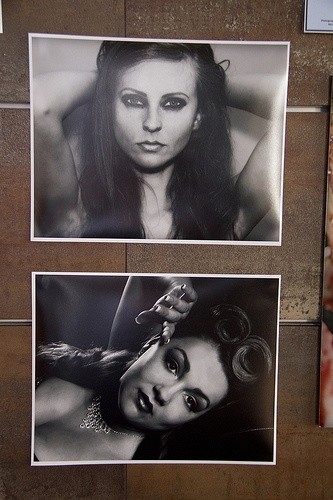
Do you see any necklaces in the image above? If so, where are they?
[80,395,143,439]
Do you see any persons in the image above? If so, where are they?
[33,276,274,461]
[33,40,280,241]
